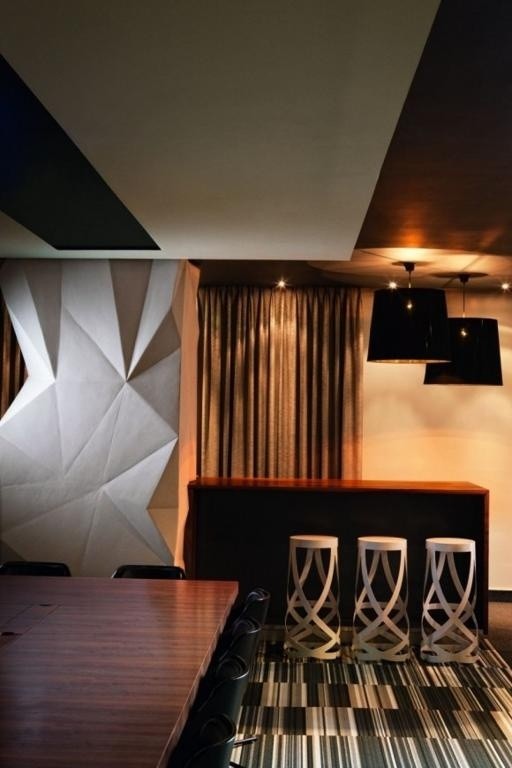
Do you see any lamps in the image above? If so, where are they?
[366,262,454,365]
[422,273,504,387]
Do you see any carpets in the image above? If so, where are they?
[227,628,511,767]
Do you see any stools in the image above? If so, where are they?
[349,535,413,663]
[281,534,343,661]
[420,535,480,666]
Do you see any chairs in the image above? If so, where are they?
[1,560,76,577]
[110,563,189,578]
[170,585,271,767]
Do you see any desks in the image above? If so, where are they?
[181,476,490,636]
[0,574,239,767]
